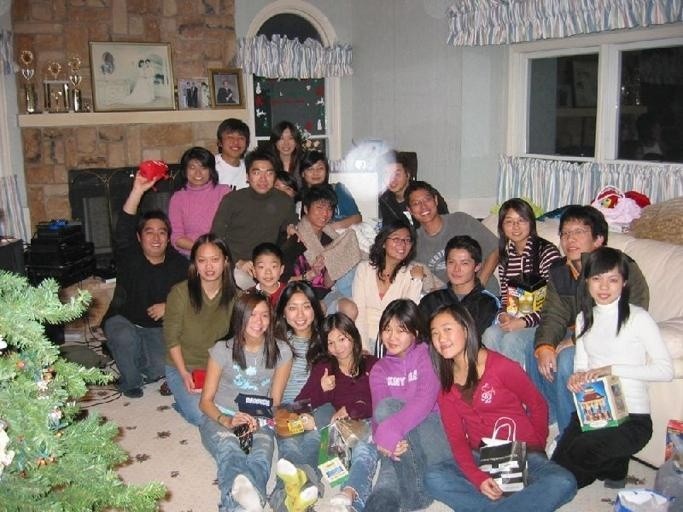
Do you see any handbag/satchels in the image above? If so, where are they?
[591,185,642,224]
[478,417,529,493]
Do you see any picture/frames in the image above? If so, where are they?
[87,39,246,113]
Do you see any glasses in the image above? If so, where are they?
[387,238,415,247]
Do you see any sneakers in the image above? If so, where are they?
[124,388,143,398]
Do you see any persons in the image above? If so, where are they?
[186,82,192,107]
[200,81,209,106]
[123,60,148,105]
[217,79,236,103]
[190,81,197,109]
[100,117,673,512]
[144,59,155,101]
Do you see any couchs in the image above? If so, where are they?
[481,212,682,470]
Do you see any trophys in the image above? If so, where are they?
[42,60,70,113]
[67,55,85,112]
[20,50,38,113]
